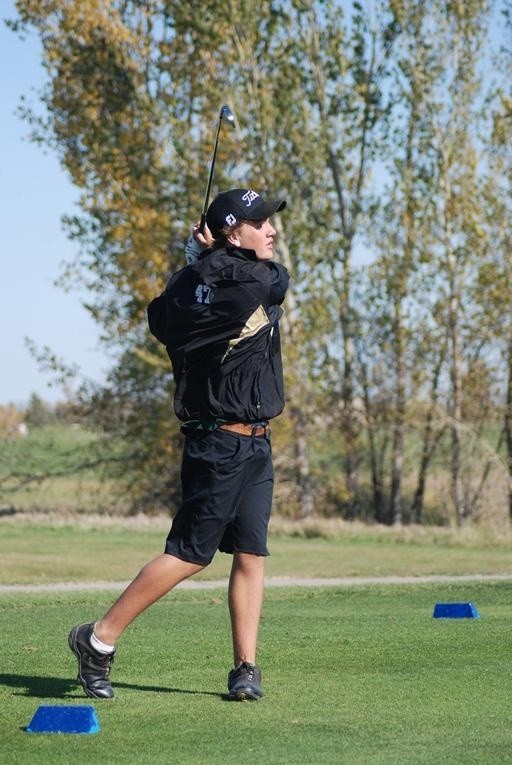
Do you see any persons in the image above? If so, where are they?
[68,186,291,702]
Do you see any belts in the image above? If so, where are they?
[220,421,271,440]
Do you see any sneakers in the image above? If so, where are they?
[69,621,117,698]
[228,662,264,700]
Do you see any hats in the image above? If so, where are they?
[206,190,287,239]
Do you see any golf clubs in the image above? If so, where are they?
[199,105,235,234]
